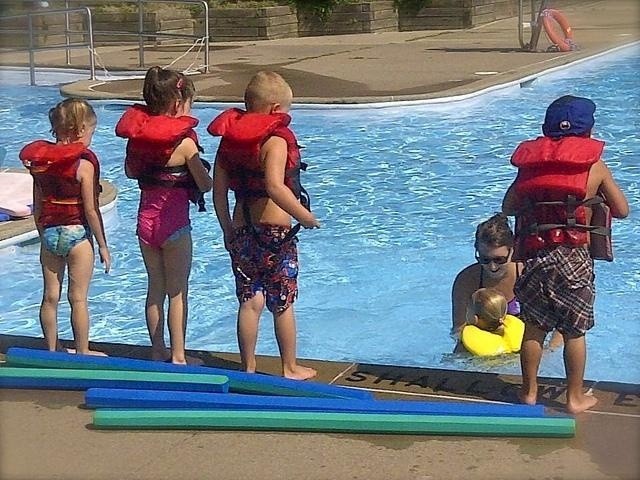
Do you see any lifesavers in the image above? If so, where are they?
[543,8,575,51]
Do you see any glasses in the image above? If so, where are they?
[474,248,511,265]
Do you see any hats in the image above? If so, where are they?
[542,96,596,137]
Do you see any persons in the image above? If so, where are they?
[502,94,630,414]
[451,211,526,340]
[212,68,318,381]
[123,66,214,366]
[31,97,112,355]
[453,286,508,354]
